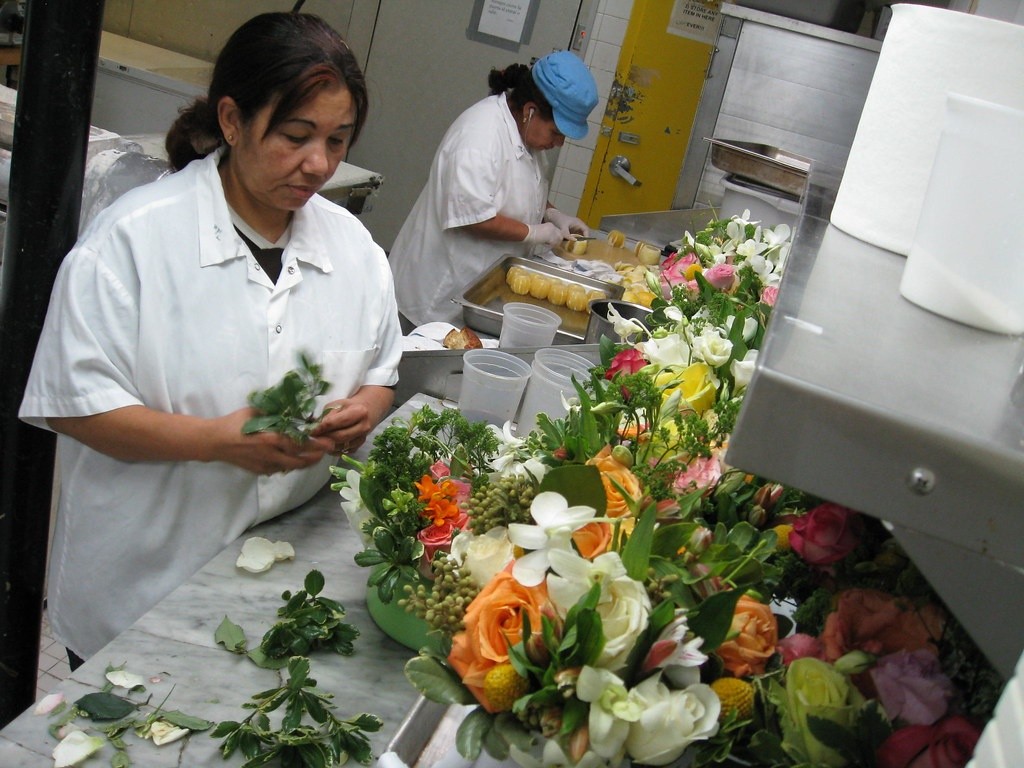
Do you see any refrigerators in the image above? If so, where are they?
[90,29,214,135]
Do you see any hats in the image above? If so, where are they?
[532,50,599,139]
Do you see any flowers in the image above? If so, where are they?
[329,207,1003,768]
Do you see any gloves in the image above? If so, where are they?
[519,221,563,247]
[543,207,589,241]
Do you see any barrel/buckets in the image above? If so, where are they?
[900,94,1024,336]
[829,2,1024,259]
[584,300,656,346]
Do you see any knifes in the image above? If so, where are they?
[562,236,596,242]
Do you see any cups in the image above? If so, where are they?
[498,302,561,365]
[457,347,532,428]
[518,347,596,441]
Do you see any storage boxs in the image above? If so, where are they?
[701,136,812,196]
[451,253,626,344]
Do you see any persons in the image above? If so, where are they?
[16,12,404,675]
[388,51,599,337]
